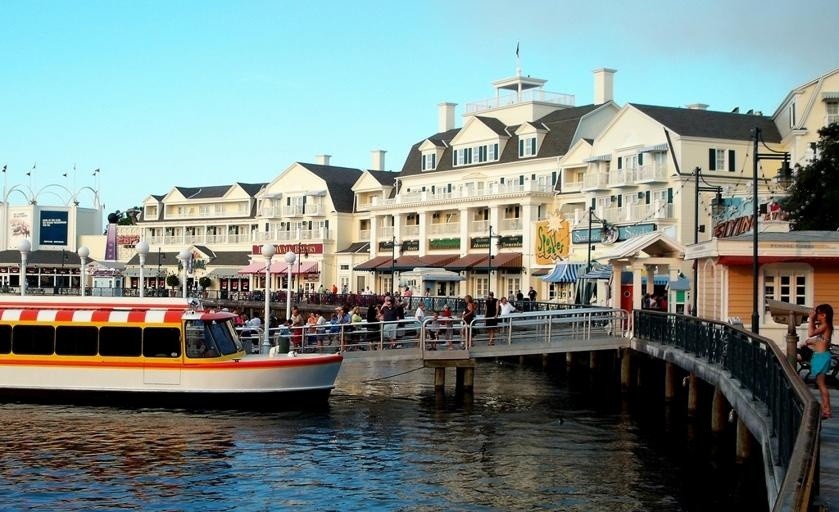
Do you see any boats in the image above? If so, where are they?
[0,295,344,410]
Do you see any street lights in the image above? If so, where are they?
[488,224,500,296]
[157,246,166,295]
[260,244,277,353]
[297,242,309,303]
[78,246,89,296]
[135,242,150,296]
[60,248,70,295]
[18,239,31,296]
[179,249,192,298]
[391,235,403,294]
[285,252,297,321]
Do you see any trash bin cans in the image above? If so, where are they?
[727,316,748,343]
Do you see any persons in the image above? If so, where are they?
[298,285,304,303]
[123,282,230,300]
[658,293,668,312]
[649,292,658,309]
[310,284,372,306]
[366,292,408,350]
[234,306,261,353]
[461,295,477,349]
[415,302,454,350]
[269,306,326,353]
[642,291,645,308]
[485,293,515,345]
[780,321,822,362]
[424,288,431,310]
[508,287,537,310]
[405,287,412,310]
[806,303,835,420]
[644,293,650,308]
[327,306,363,351]
